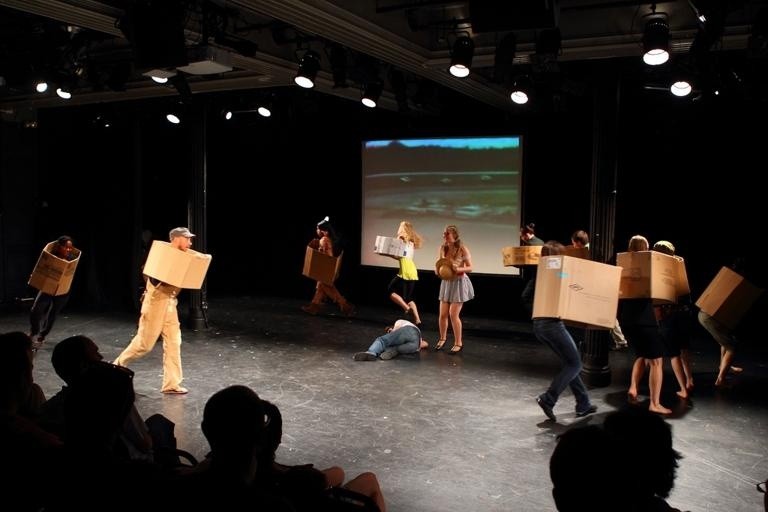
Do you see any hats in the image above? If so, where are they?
[436,258,457,280]
[170,227,195,239]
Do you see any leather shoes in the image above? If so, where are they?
[434,338,446,350]
[448,344,465,354]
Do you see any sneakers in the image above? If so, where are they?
[536,396,555,421]
[576,405,598,413]
[353,352,376,361]
[380,351,398,360]
[301,306,315,315]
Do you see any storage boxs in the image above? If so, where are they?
[671,254,691,298]
[303,238,345,286]
[141,239,211,291]
[374,235,416,258]
[696,266,744,317]
[529,254,623,329]
[613,250,680,305]
[29,239,82,297]
[551,240,590,260]
[501,245,542,267]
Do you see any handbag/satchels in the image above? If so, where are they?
[145,413,197,468]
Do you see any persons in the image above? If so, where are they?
[519,240,599,421]
[569,231,629,353]
[616,235,674,415]
[103,226,197,395]
[1,329,391,512]
[518,223,546,319]
[352,319,429,361]
[430,224,475,356]
[378,221,425,331]
[547,240,766,398]
[25,234,79,358]
[302,220,362,324]
[548,409,674,512]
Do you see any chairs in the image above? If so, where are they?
[160,442,203,467]
[309,484,384,511]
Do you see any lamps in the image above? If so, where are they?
[637,3,673,68]
[663,52,695,99]
